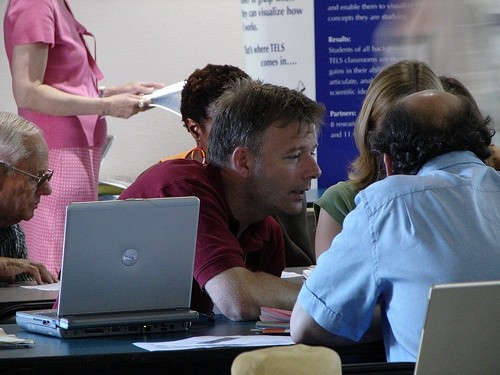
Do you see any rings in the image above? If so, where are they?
[139,101,144,109]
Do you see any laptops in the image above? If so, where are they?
[414,280,500,375]
[16,195,201,339]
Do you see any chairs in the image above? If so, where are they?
[341,280,500,375]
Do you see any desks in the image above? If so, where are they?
[0,314,289,375]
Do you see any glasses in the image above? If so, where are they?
[7,166,54,187]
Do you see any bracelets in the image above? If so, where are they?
[98,86,105,97]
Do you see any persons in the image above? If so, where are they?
[0,112,59,285]
[313,59,445,265]
[439,75,500,171]
[160,64,317,268]
[290,90,500,363]
[4,0,166,281]
[51,78,325,322]
